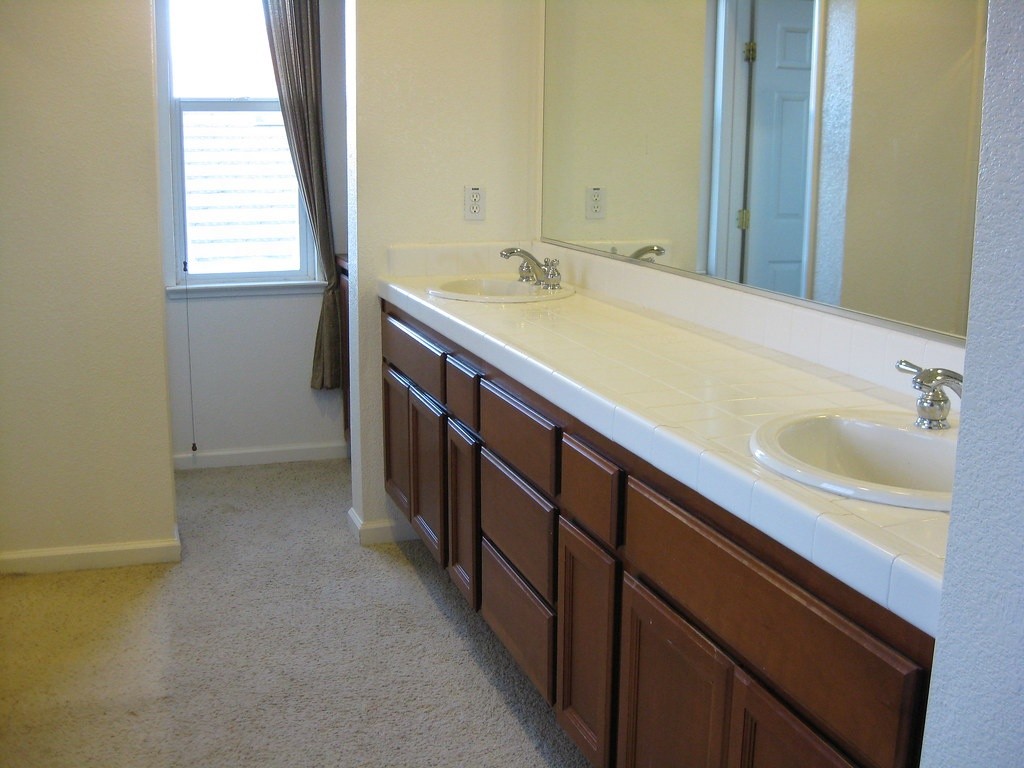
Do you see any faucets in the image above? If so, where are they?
[626,244,666,260]
[898,361,964,429]
[499,247,551,286]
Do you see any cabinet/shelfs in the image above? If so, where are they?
[373,265,961,768]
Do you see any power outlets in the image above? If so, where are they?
[585,186,606,220]
[464,184,488,220]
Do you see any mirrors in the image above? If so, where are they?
[538,0,987,340]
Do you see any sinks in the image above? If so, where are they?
[749,406,961,513]
[427,272,577,304]
